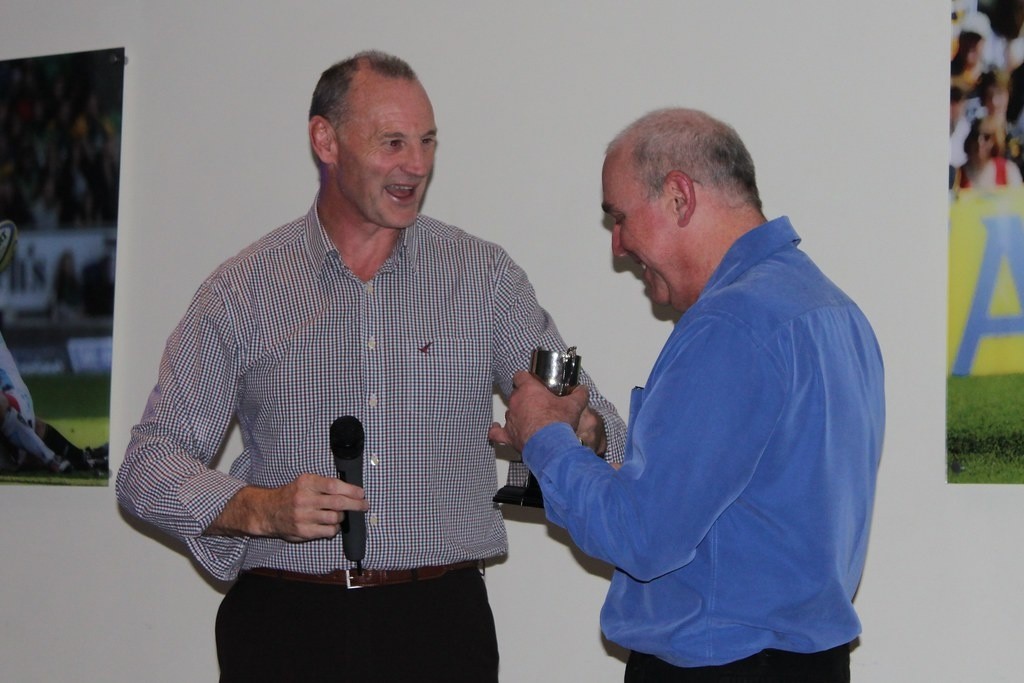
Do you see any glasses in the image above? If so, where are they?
[970,132,993,141]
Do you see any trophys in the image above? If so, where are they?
[491,346,582,507]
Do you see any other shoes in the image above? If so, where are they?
[45,453,70,474]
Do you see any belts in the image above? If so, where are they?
[252,558,479,589]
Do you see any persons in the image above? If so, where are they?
[487,107,886,683]
[47,239,117,318]
[0,334,110,477]
[0,60,120,231]
[116,51,627,683]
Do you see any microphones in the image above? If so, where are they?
[329,414,367,576]
